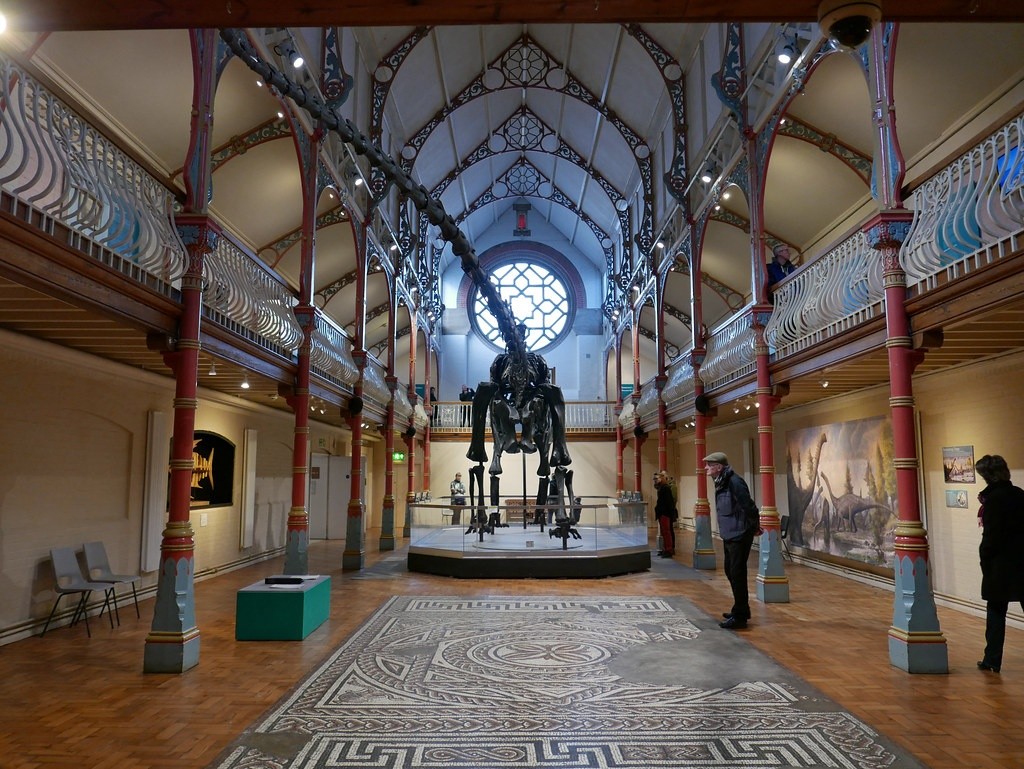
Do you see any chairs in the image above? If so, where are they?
[40,541,141,639]
[779,515,793,562]
[441,508,454,526]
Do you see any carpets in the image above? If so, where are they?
[198,594,934,769]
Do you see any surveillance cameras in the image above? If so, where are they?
[817,1,883,56]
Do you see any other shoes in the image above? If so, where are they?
[976,660,1001,673]
[656,549,676,559]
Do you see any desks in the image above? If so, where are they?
[235,574,331,641]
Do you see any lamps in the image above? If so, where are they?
[819,372,828,388]
[778,32,802,64]
[273,43,304,69]
[311,400,326,414]
[633,275,645,290]
[381,240,397,251]
[424,307,435,323]
[702,160,720,183]
[208,359,216,376]
[685,416,695,428]
[609,305,623,321]
[239,373,250,388]
[344,169,362,185]
[657,237,668,249]
[733,396,760,414]
[408,278,418,292]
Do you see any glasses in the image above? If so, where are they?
[653,477,660,481]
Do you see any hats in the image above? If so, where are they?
[702,451,728,462]
[455,472,461,477]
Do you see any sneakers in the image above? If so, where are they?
[722,607,751,619]
[719,615,748,629]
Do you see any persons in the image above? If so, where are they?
[459,384,475,427]
[976,454,1024,673]
[653,470,678,558]
[430,387,438,427]
[702,452,764,630]
[451,473,466,526]
[766,239,801,306]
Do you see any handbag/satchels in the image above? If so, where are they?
[455,497,465,506]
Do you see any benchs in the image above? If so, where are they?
[505,499,548,524]
